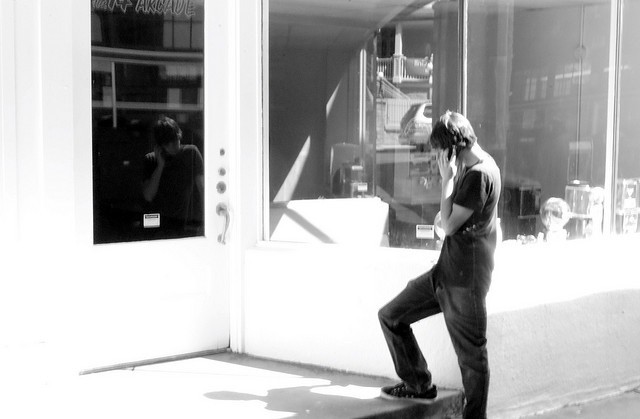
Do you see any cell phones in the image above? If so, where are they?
[448,145,461,161]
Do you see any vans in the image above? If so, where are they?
[397,102,433,150]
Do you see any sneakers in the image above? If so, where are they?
[380,382,439,404]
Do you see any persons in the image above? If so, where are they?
[377,109,503,419]
[137,116,204,241]
